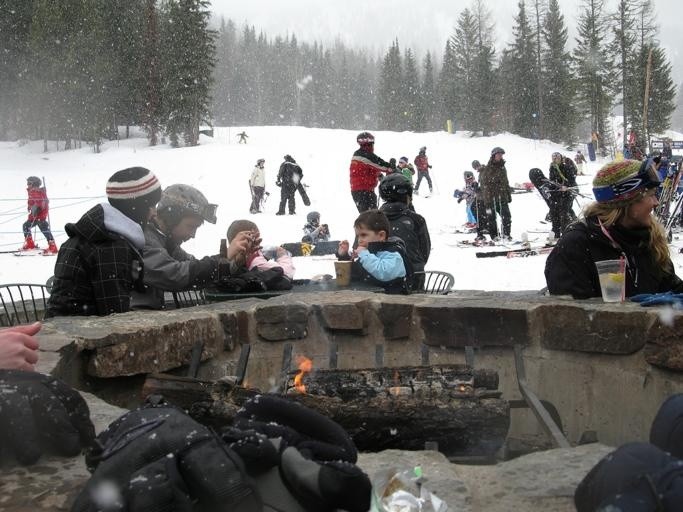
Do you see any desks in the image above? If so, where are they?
[204,278,386,298]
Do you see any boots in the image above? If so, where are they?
[44,243,57,253]
[23,236,35,249]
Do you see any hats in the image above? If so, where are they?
[592,159,647,209]
[464,171,472,180]
[398,157,408,164]
[106,166,161,209]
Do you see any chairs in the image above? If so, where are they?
[170,275,207,307]
[406,270,454,295]
[1,283,54,328]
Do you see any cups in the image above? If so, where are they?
[595,260,628,302]
[334,260,354,286]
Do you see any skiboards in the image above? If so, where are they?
[527,228,552,233]
[655,177,683,237]
[477,247,554,258]
[457,240,522,247]
[248,179,257,212]
[0,245,59,256]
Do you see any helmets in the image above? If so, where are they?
[307,212,319,226]
[356,132,374,143]
[158,183,208,219]
[378,173,413,203]
[26,176,41,187]
[490,147,504,155]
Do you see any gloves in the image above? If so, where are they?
[230,266,283,292]
[190,385,358,465]
[72,404,279,511]
[0,368,96,466]
[32,206,40,217]
[630,291,682,307]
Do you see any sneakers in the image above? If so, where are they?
[465,222,512,242]
[545,240,556,247]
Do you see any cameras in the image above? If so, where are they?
[247,233,256,249]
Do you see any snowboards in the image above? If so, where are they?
[529,169,556,208]
[297,182,311,206]
[280,241,346,258]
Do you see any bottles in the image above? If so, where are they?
[216,240,231,279]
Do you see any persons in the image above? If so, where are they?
[334,210,415,295]
[456,147,515,242]
[546,149,588,239]
[622,141,678,214]
[226,219,295,289]
[128,185,262,311]
[0,319,40,371]
[378,173,432,291]
[41,167,162,317]
[544,159,682,300]
[248,154,304,216]
[22,176,58,255]
[236,132,248,145]
[302,211,332,242]
[349,131,433,213]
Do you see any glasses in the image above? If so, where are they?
[184,198,217,224]
[637,157,661,189]
